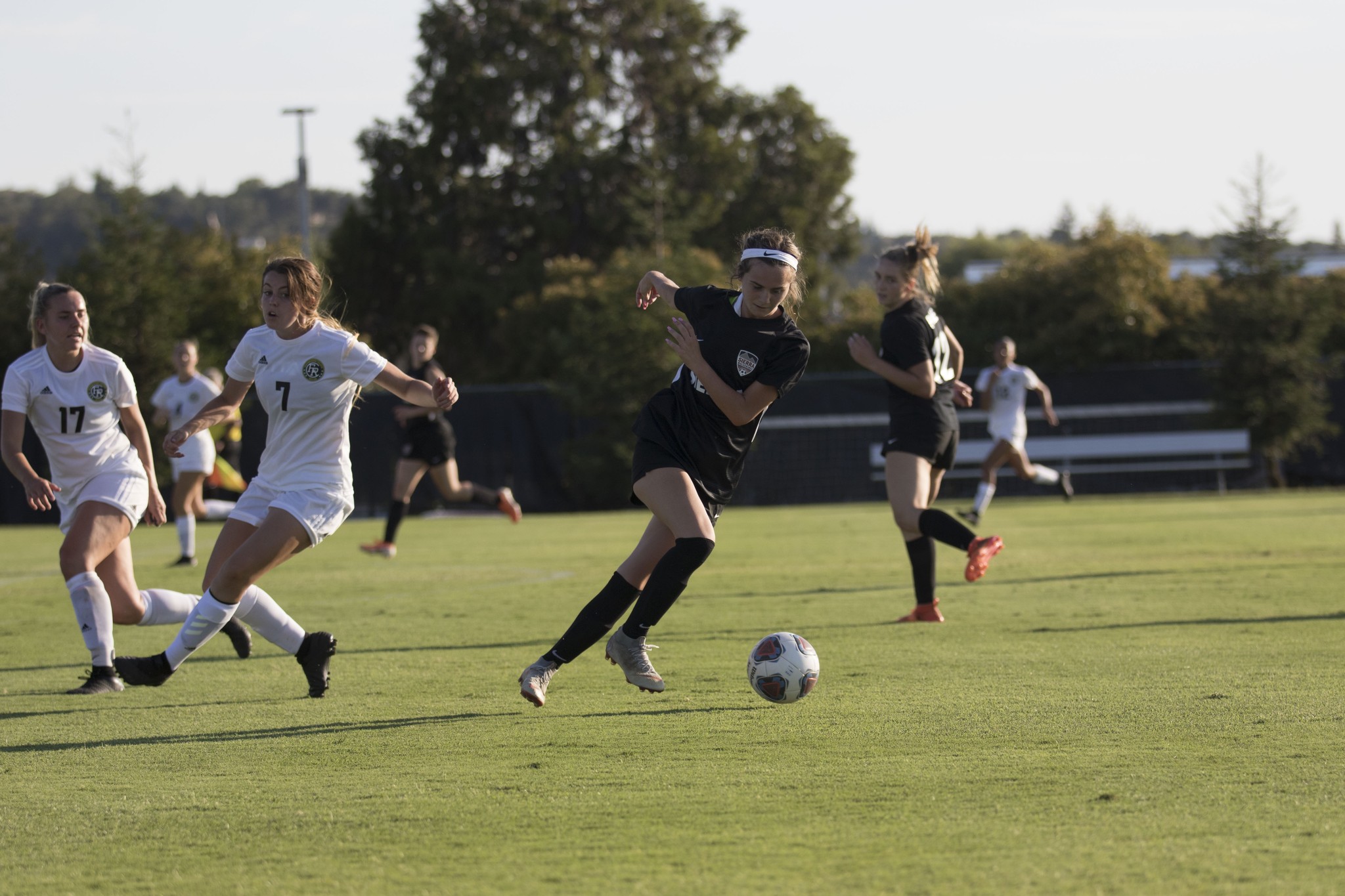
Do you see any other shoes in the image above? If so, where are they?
[1058,472,1074,499]
[360,542,396,560]
[497,487,521,521]
[960,511,978,526]
[167,554,198,569]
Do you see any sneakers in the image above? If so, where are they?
[898,597,945,623]
[605,623,666,694]
[965,535,1004,583]
[66,666,125,697]
[295,631,338,698]
[517,655,559,709]
[112,651,178,688]
[217,615,252,658]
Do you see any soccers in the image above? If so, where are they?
[746,632,820,704]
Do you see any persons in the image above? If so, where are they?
[0,283,250,694]
[954,331,1075,527]
[848,224,1005,623]
[109,249,460,698]
[358,324,524,561]
[201,366,247,494]
[517,227,810,708]
[150,336,237,569]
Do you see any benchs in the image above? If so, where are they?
[868,431,1252,493]
